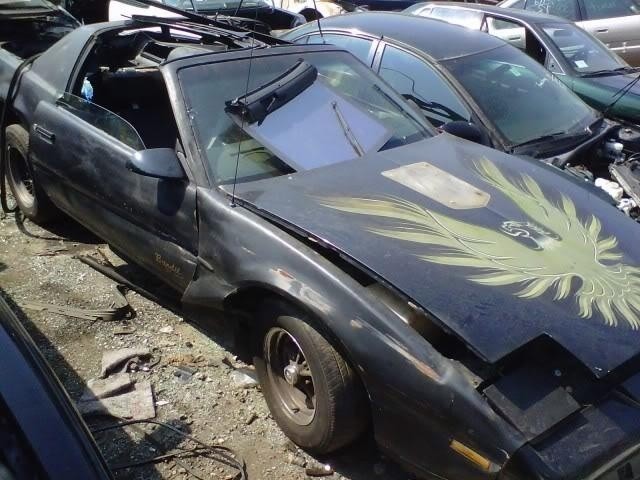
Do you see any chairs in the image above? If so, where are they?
[87,59,269,142]
